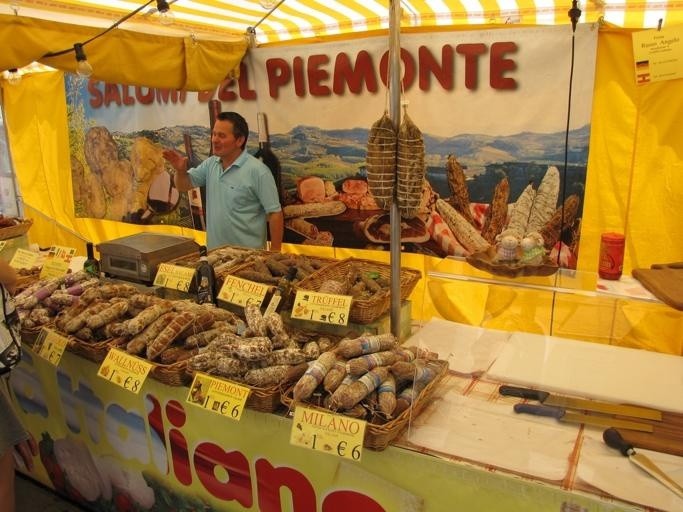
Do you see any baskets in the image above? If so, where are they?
[160,244,258,298]
[290,256,423,326]
[186,327,343,413]
[279,332,451,453]
[105,310,248,387]
[46,286,200,364]
[8,274,58,347]
[220,252,341,311]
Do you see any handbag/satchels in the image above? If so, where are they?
[1,282,22,378]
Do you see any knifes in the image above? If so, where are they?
[602,424,683,500]
[498,383,664,422]
[513,400,652,432]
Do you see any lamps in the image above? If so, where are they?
[157,0,175,25]
[74,44,94,79]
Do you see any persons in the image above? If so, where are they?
[0,257,29,512]
[161,111,284,252]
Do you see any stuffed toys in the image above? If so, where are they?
[520,233,545,265]
[492,230,521,264]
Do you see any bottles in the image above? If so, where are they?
[195,246,217,308]
[597,231,626,282]
[84,242,100,279]
[259,266,297,319]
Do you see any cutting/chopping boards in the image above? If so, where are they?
[608,401,682,455]
[632,267,683,311]
